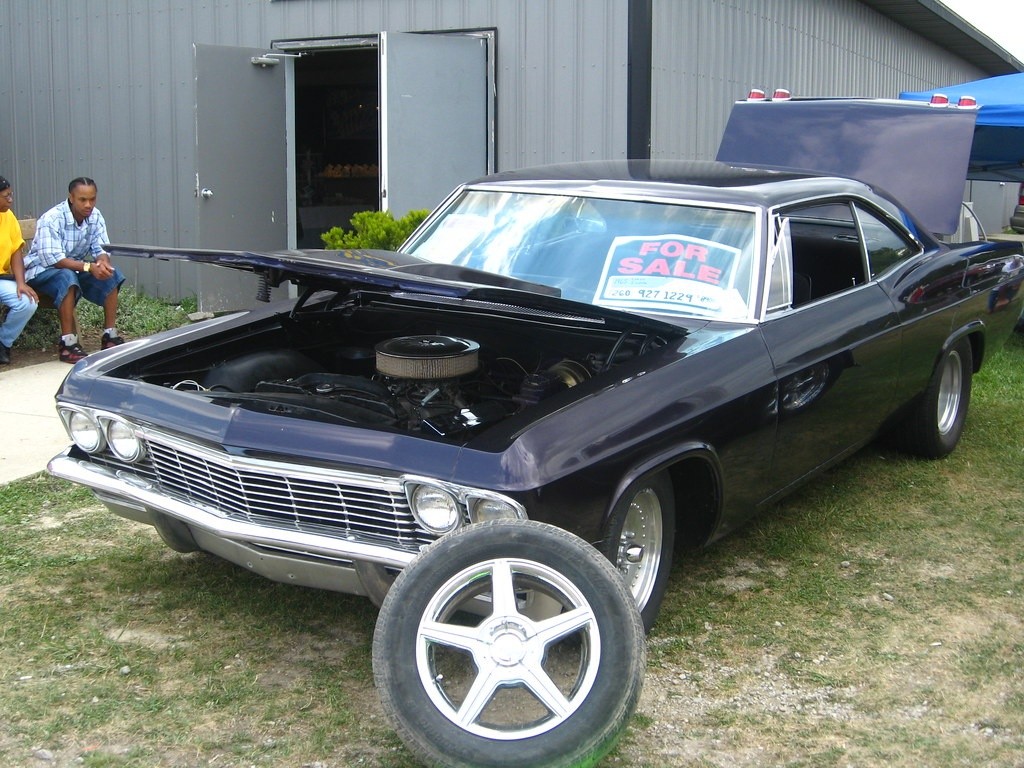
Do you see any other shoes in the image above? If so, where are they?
[0,341,10,364]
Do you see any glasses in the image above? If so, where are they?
[0,191,14,200]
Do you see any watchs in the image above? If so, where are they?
[83,262,91,272]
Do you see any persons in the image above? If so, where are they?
[24,177,128,364]
[0,176,39,364]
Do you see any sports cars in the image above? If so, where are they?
[42,87,1024,638]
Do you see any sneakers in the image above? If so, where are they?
[59,334,88,364]
[101,328,125,350]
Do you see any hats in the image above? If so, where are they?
[0,175,10,191]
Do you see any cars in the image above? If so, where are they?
[1008,185,1024,234]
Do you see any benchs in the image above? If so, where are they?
[16,218,85,349]
